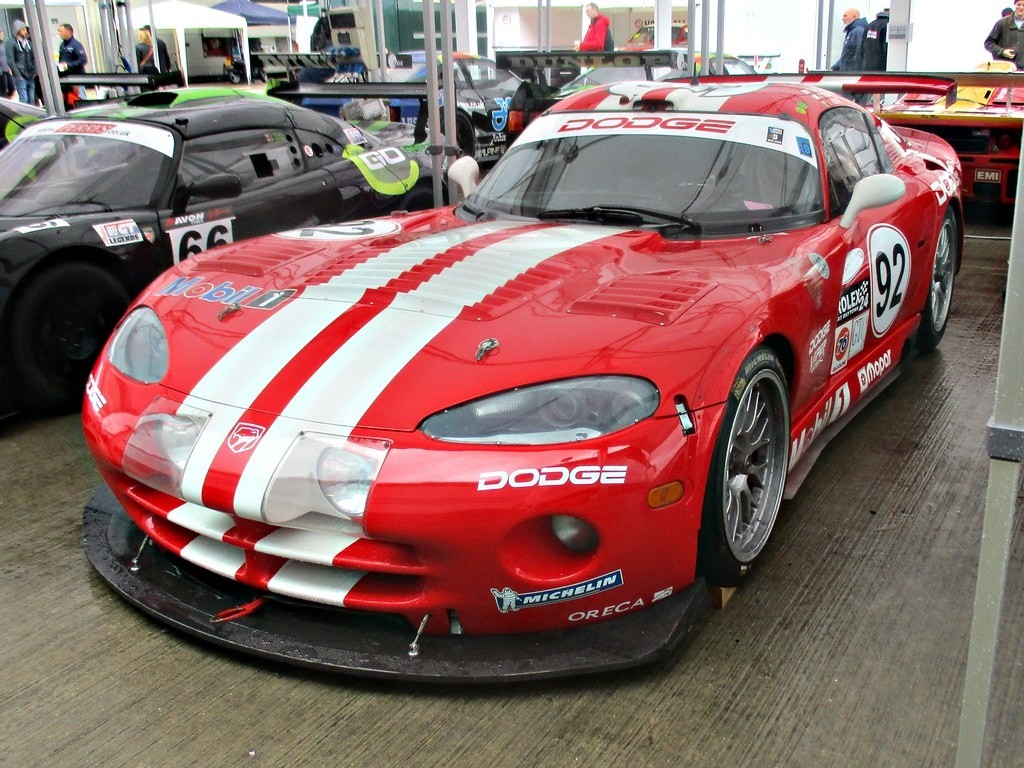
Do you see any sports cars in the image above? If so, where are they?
[221,16,1024,260]
[79,68,966,689]
[0,66,470,420]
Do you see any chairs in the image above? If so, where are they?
[716,146,791,213]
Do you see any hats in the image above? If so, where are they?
[139,25,150,33]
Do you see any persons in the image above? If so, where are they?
[0,18,170,114]
[204,39,225,55]
[832,0,1024,71]
[574,3,611,52]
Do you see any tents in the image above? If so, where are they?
[124,0,295,89]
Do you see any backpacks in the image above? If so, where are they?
[604,25,614,60]
